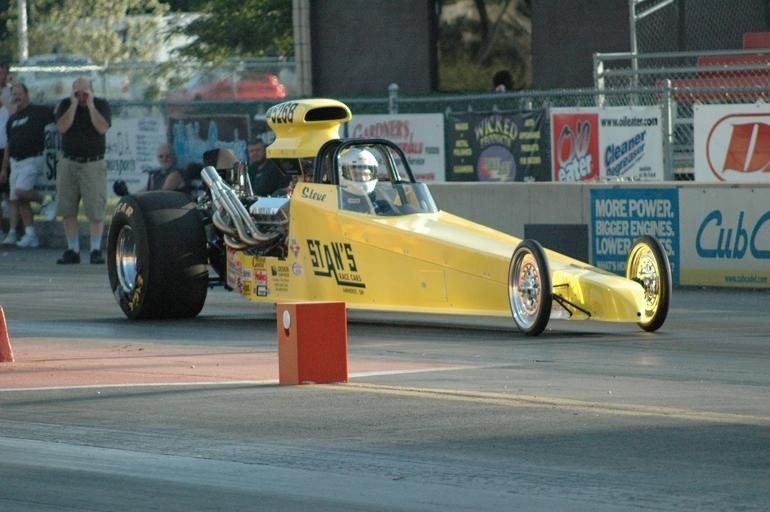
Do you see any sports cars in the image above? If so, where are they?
[107,98,672,337]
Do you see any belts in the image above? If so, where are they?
[62,153,104,163]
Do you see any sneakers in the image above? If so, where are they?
[0,229,20,246]
[88,249,106,265]
[55,248,84,264]
[15,232,41,248]
[46,200,59,223]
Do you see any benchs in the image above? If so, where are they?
[655,32,770,102]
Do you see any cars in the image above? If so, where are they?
[166,72,286,99]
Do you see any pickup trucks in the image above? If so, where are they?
[8,52,130,102]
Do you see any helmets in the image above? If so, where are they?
[335,145,380,197]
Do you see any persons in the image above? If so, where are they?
[245,136,279,195]
[1,63,19,115]
[493,68,514,91]
[338,146,381,213]
[51,78,113,265]
[0,99,20,246]
[135,142,186,192]
[0,83,56,248]
